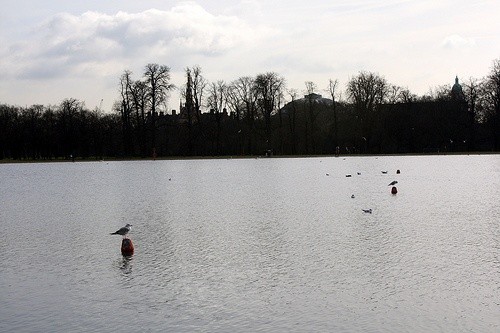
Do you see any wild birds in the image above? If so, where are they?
[110,224,133,239]
[362,209,372,213]
[388,180,398,186]
